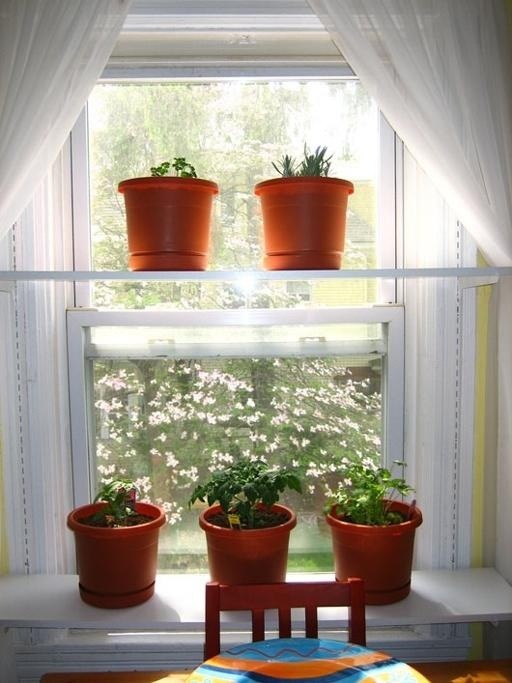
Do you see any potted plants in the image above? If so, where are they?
[188,456,303,583]
[65,479,166,610]
[324,460,423,607]
[255,142,354,270]
[118,157,219,270]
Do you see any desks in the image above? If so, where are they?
[39,658,512,683]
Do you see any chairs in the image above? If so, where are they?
[203,577,366,662]
